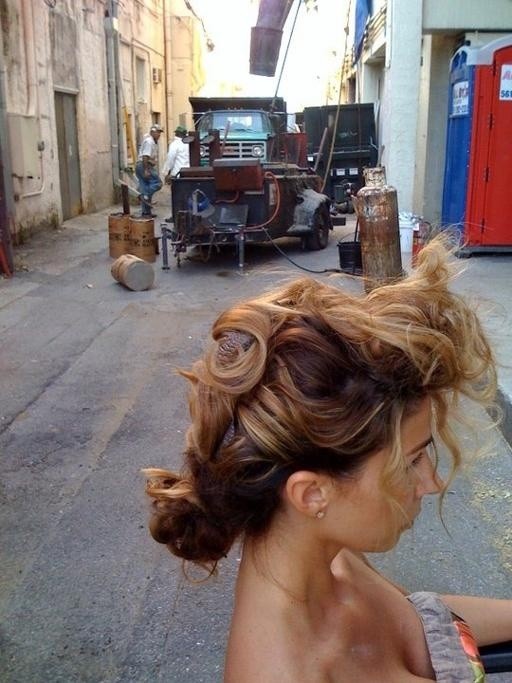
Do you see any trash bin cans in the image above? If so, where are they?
[338,240,363,272]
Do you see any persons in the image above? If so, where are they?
[162,124,191,222]
[134,123,164,219]
[138,220,510,682]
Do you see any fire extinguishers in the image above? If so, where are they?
[411,216,432,269]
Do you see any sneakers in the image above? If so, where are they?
[140,194,154,208]
[165,216,174,222]
[141,212,157,218]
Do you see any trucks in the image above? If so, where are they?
[188,96,291,170]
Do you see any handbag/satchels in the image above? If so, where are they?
[165,174,177,185]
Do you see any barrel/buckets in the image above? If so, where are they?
[335,230,363,275]
[128,216,156,263]
[400,220,414,252]
[108,215,134,258]
[109,252,155,291]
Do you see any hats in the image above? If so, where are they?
[150,123,164,133]
[173,126,188,134]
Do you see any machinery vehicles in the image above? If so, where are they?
[160,128,348,277]
